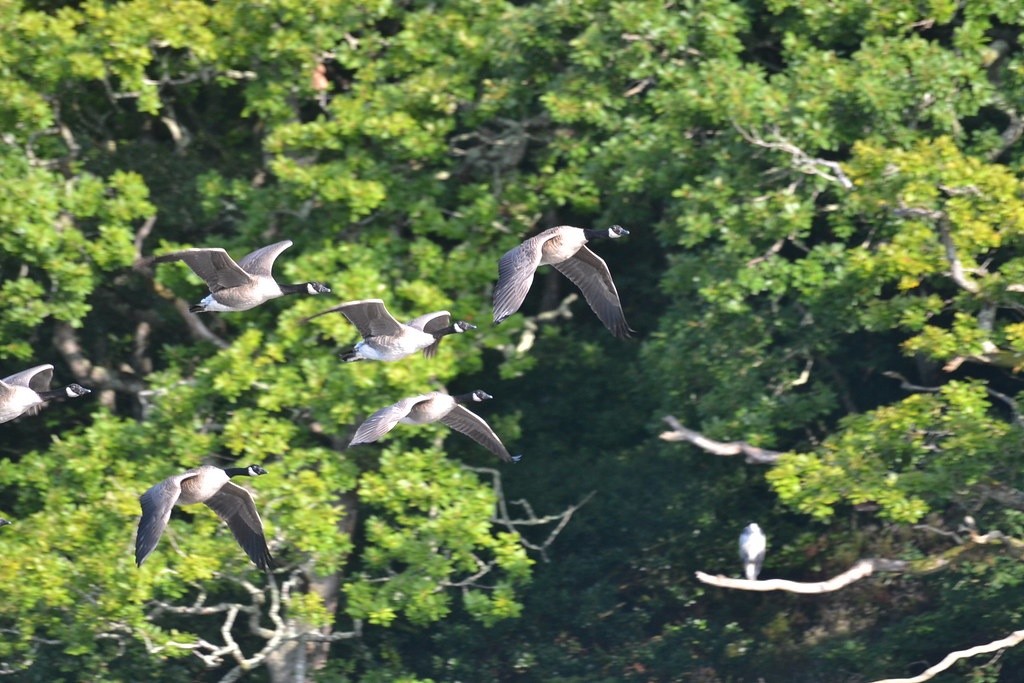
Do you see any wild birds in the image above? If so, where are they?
[0,519,11,534]
[297,299,478,365]
[738,522,766,582]
[492,224,634,344]
[134,464,277,573]
[132,239,332,313]
[0,364,91,426]
[348,389,521,464]
